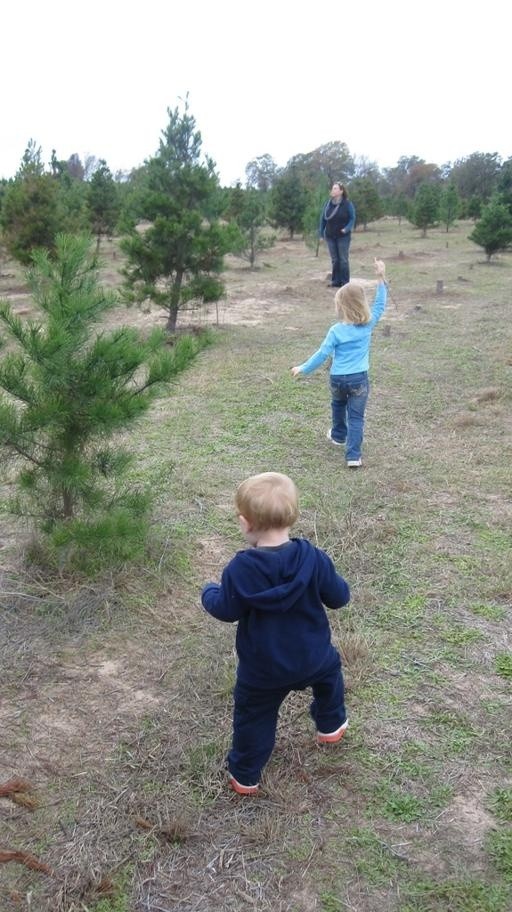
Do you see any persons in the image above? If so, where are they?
[291,255,387,469]
[319,184,357,289]
[200,471,352,792]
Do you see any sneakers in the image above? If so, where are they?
[347,457,361,467]
[227,771,259,794]
[328,428,347,445]
[316,718,349,743]
